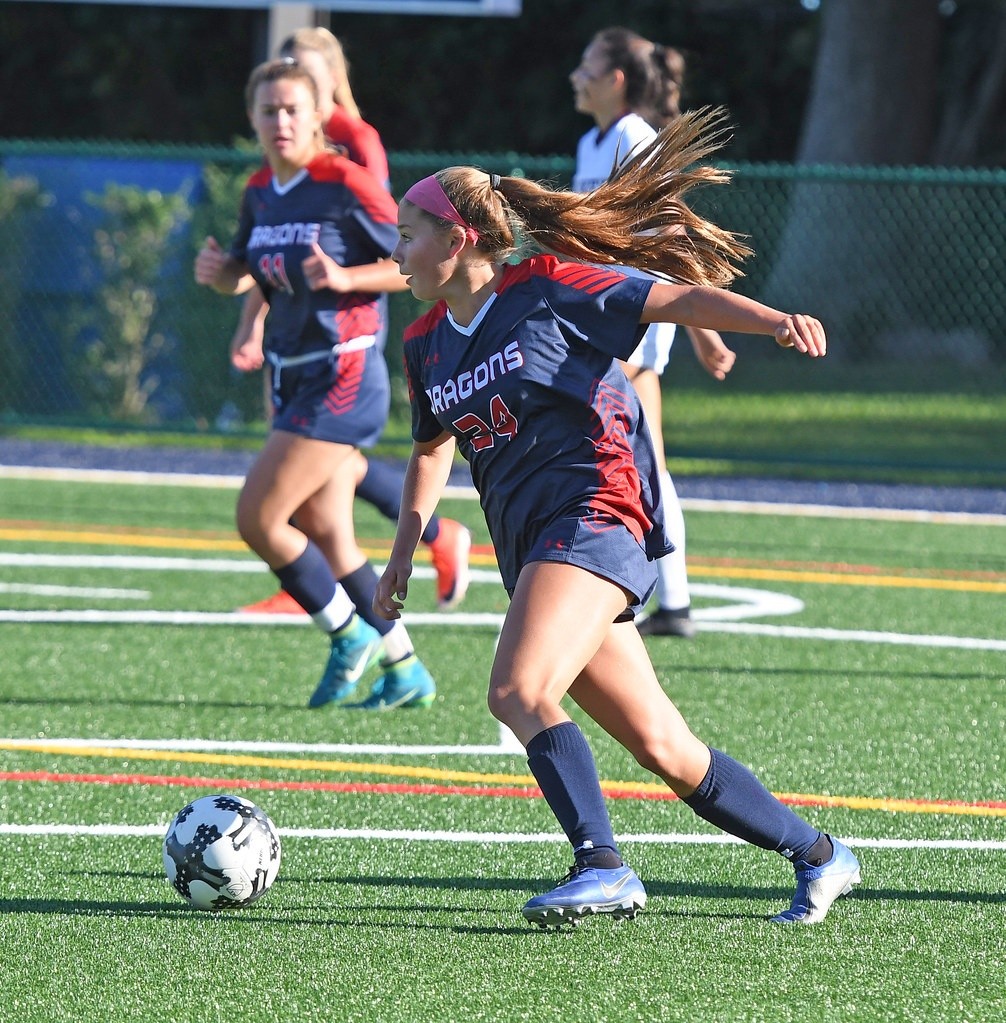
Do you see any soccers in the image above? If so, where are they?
[162,794,281,911]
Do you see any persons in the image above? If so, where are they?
[572,28,737,639]
[197,60,435,708]
[372,106,862,928]
[233,29,470,615]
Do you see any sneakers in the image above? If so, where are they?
[234,586,307,616]
[423,517,472,609]
[520,858,646,930]
[303,612,387,713]
[343,650,439,716]
[767,833,862,927]
[633,603,694,637]
